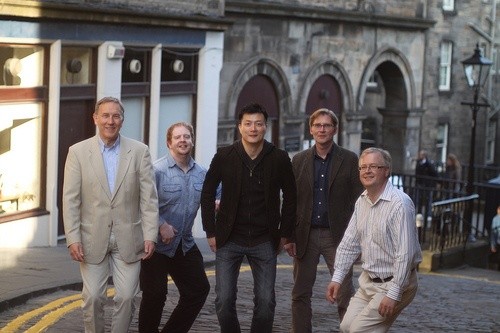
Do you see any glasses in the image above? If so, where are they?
[312,122,337,129]
[358,164,388,171]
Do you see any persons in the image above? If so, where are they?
[138,122,222,333]
[416,150,500,271]
[284,108,366,333]
[63,97,159,333]
[200,103,297,333]
[327,147,423,333]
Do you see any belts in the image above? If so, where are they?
[369,275,395,283]
